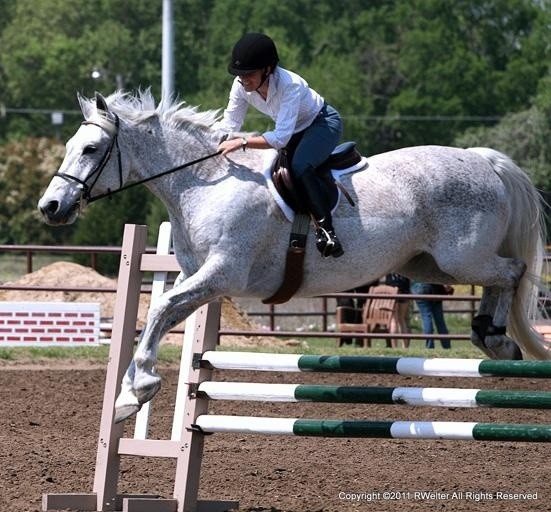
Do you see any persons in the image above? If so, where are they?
[384,271,410,348]
[211,32,344,258]
[337,279,379,346]
[412,281,454,349]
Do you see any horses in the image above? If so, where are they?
[36,84,551,425]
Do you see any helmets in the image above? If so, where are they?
[227,33,279,77]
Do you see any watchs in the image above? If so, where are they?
[241,136,248,152]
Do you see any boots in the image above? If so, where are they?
[293,168,344,258]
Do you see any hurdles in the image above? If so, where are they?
[41,222,551,512]
